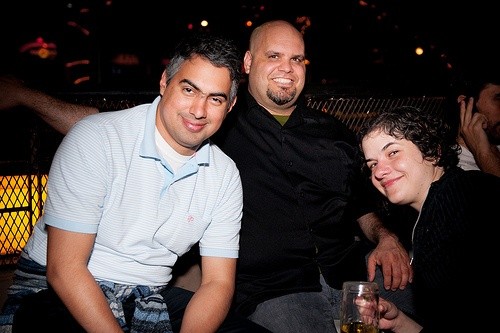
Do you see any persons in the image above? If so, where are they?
[1,22,413,333]
[459,95,499,176]
[453,82,500,171]
[354,107,500,332]
[13,38,242,332]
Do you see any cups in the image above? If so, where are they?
[341,282,379,333]
